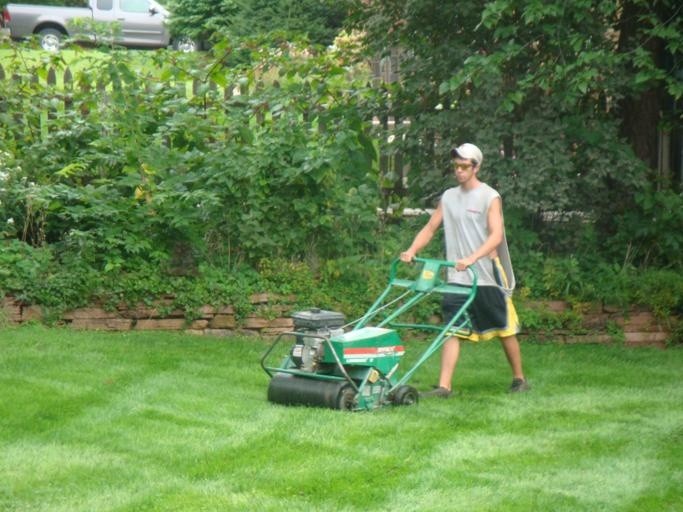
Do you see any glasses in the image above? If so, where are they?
[453,161,475,171]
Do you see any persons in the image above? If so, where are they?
[398,141,529,398]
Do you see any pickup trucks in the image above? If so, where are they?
[2,0,202,51]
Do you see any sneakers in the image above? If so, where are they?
[419,387,453,399]
[506,376,528,394]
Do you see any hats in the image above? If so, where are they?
[451,143,483,166]
